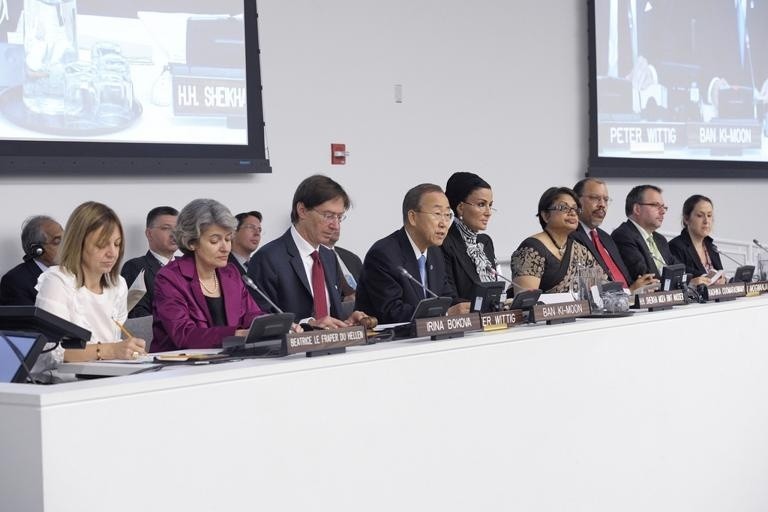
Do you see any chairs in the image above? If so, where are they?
[0,336,36,383]
[121,315,153,353]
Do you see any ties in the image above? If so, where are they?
[418,256,427,299]
[591,230,628,288]
[311,251,328,319]
[648,237,664,269]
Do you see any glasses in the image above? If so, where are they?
[548,205,584,215]
[583,194,612,204]
[464,200,498,213]
[639,201,668,212]
[313,208,347,223]
[244,225,262,234]
[419,209,453,220]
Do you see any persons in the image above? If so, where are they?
[149,199,305,353]
[0,214,66,306]
[28,201,147,383]
[354,183,472,324]
[246,175,369,330]
[511,186,611,298]
[440,171,497,312]
[571,178,660,293]
[611,185,721,292]
[228,211,261,276]
[669,195,726,286]
[121,206,180,319]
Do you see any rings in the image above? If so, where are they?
[132,351,139,359]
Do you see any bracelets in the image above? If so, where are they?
[96,341,102,361]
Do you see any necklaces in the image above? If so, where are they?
[545,229,567,256]
[198,274,218,294]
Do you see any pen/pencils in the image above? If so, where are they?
[111,317,149,356]
[649,276,652,280]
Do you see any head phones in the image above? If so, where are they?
[22,242,45,263]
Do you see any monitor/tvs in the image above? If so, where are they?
[588,0,768,180]
[0,0,275,177]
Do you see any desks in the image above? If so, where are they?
[0,291,768,511]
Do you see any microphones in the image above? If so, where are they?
[485,261,529,292]
[751,237,768,254]
[646,253,665,265]
[711,244,742,266]
[242,271,298,312]
[395,263,441,300]
